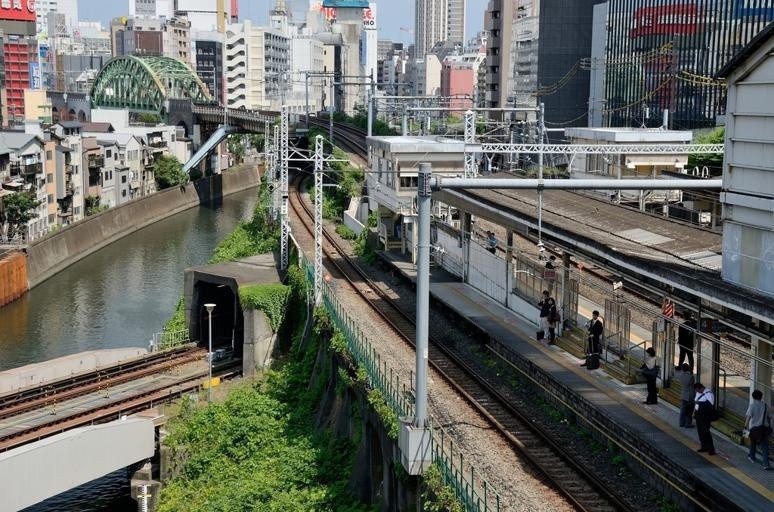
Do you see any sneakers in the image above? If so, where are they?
[760,463,769,469]
[748,454,755,463]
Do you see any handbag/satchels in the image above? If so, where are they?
[750,425,768,443]
[547,312,560,321]
[640,363,659,377]
[537,331,544,340]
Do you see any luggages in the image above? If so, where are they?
[586,336,599,369]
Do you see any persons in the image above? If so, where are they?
[485,231,497,254]
[545,255,557,297]
[580,310,604,367]
[743,389,769,470]
[677,312,695,374]
[547,297,557,345]
[538,290,550,339]
[694,383,714,455]
[678,363,695,427]
[642,347,658,405]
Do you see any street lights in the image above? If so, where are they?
[203,303,217,402]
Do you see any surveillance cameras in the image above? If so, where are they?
[613,281,622,289]
